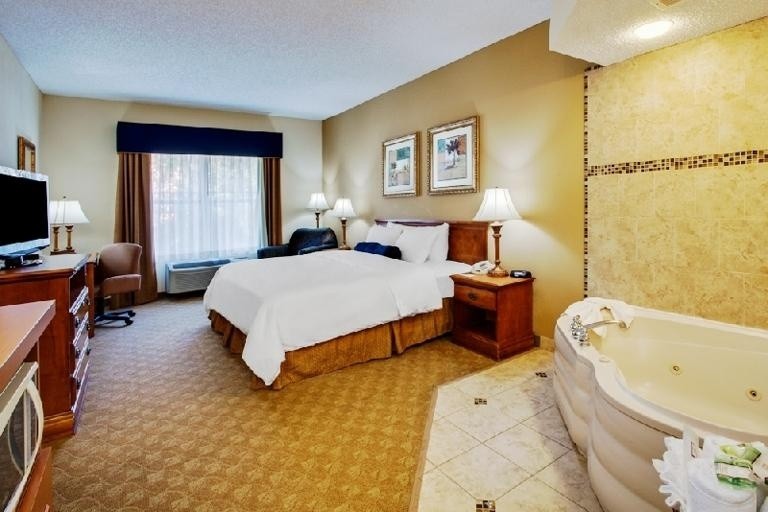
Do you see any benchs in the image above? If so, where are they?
[165,257,248,294]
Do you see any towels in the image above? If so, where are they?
[565,297,635,338]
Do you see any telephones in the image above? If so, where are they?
[471,260,496,275]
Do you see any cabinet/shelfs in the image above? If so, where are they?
[0,252,96,512]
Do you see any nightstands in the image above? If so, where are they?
[448,273,536,362]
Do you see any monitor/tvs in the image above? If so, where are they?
[0,166,50,269]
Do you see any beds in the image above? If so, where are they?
[203,219,493,390]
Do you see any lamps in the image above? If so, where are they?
[472,187,523,276]
[305,193,356,250]
[48,201,89,255]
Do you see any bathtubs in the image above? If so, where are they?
[553,297,768,512]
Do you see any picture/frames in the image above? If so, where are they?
[18,136,36,173]
[426,115,481,197]
[380,131,421,199]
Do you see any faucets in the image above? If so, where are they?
[570,319,626,339]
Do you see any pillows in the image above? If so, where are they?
[354,220,450,264]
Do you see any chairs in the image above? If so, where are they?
[94,243,143,324]
[257,227,338,260]
